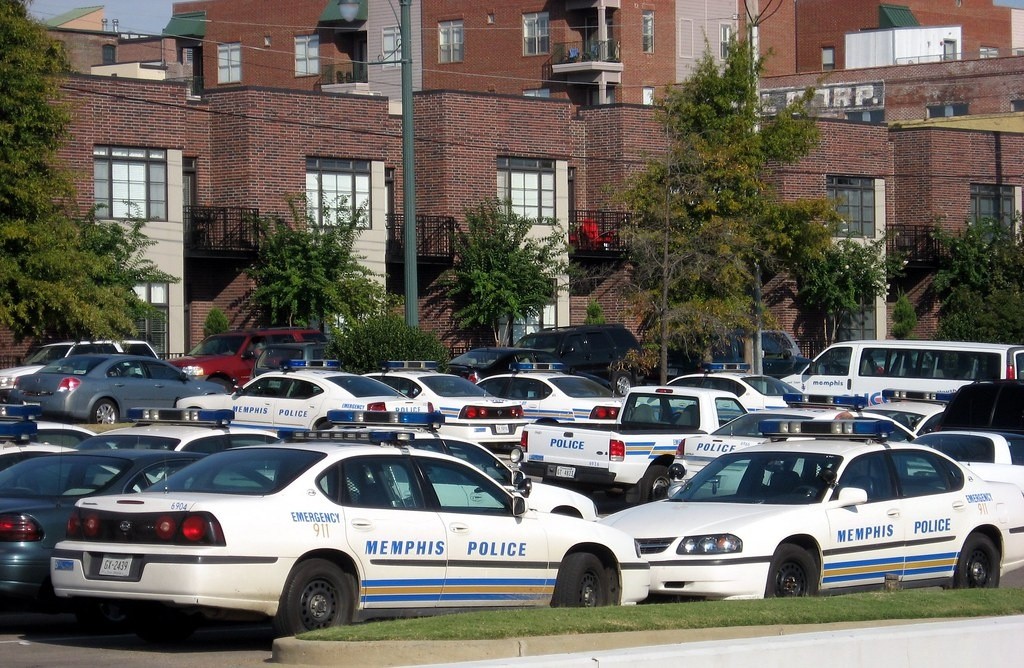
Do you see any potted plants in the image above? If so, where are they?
[569,222,579,244]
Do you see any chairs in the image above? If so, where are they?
[122,366,134,376]
[674,404,700,426]
[849,475,877,501]
[583,218,610,250]
[85,464,112,488]
[630,404,658,423]
[769,471,798,485]
[358,465,401,505]
[861,354,979,379]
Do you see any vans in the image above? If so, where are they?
[933,380,1024,441]
[777,339,1024,410]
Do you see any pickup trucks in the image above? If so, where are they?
[510,385,759,506]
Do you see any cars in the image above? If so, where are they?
[909,430,1024,484]
[599,419,1023,600]
[13,353,230,425]
[0,451,278,615]
[46,427,650,639]
[176,349,652,445]
[289,410,597,524]
[649,329,814,379]
[861,399,949,437]
[641,363,809,426]
[64,424,331,499]
[664,394,921,502]
[0,400,159,497]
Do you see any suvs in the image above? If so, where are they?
[0,337,161,404]
[514,321,645,397]
[164,327,330,397]
